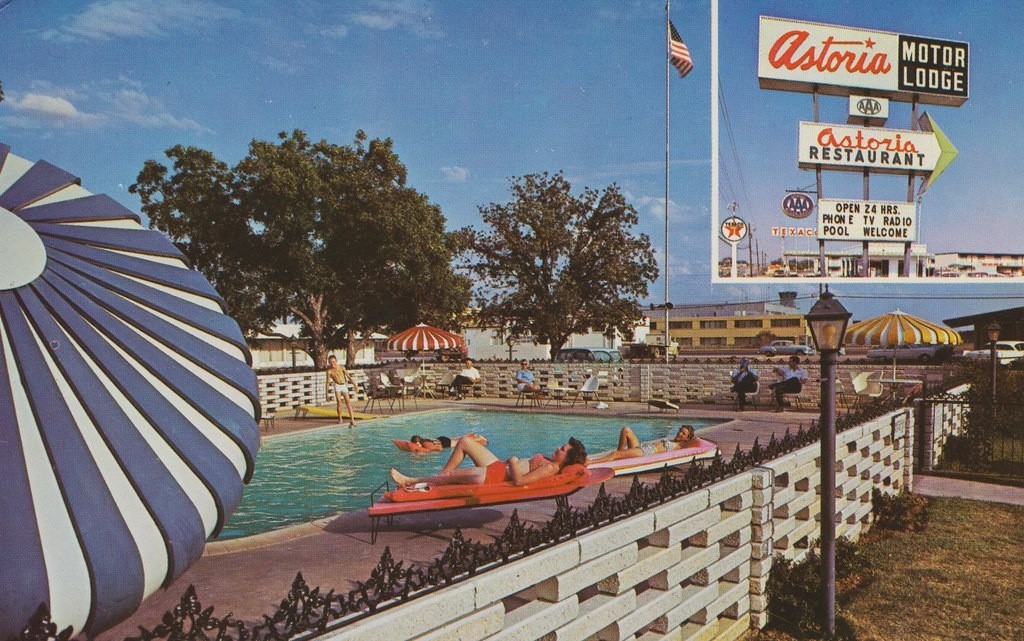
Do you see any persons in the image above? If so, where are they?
[326,355,358,425]
[391,437,590,486]
[447,358,481,400]
[731,357,759,412]
[517,359,543,398]
[586,424,697,464]
[768,356,807,413]
[412,434,487,450]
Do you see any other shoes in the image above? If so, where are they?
[449,389,454,395]
[775,407,783,413]
[769,384,774,389]
[455,397,462,401]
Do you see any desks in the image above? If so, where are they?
[373,382,402,410]
[542,387,576,409]
[412,373,443,400]
[867,378,924,406]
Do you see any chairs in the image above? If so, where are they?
[848,369,882,411]
[574,375,602,411]
[259,411,275,431]
[363,367,479,414]
[515,375,542,409]
[732,369,762,409]
[835,374,850,412]
[775,383,803,411]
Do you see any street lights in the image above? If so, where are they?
[981,316,1003,400]
[506,335,517,362]
[803,282,854,640]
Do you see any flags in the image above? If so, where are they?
[669,19,693,78]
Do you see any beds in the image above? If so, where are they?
[367,434,720,539]
[294,403,388,421]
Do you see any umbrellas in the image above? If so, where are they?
[842,310,961,398]
[0,143,263,641]
[382,321,464,397]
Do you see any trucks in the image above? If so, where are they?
[622,333,679,359]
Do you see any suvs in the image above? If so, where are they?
[554,347,630,364]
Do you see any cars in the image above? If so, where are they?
[436,348,466,362]
[962,340,1024,366]
[867,344,953,364]
[758,340,816,358]
[933,271,1008,278]
[774,270,816,277]
